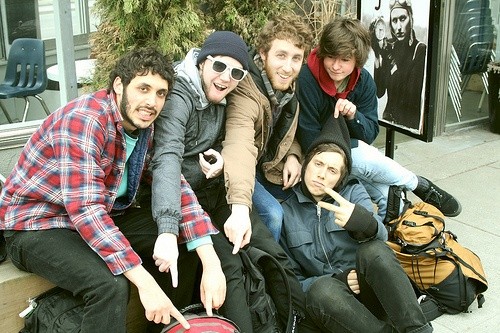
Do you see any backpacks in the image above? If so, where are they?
[240,246,297,333]
[18,286,85,333]
[383,182,491,315]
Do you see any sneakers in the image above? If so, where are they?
[415,176,462,216]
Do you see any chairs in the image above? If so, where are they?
[447,0,498,123]
[0,38,51,123]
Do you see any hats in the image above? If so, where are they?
[193,31,250,70]
[305,111,353,173]
[389,0,414,46]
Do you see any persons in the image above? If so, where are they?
[294,16,463,220]
[0,50,227,333]
[220,15,305,255]
[369,0,426,131]
[280,134,434,333]
[153,32,284,288]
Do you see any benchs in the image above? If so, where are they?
[0,254,152,333]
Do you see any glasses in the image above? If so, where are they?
[207,54,248,80]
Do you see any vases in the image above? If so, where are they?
[488,71,500,135]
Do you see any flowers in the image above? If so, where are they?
[487,59,500,74]
